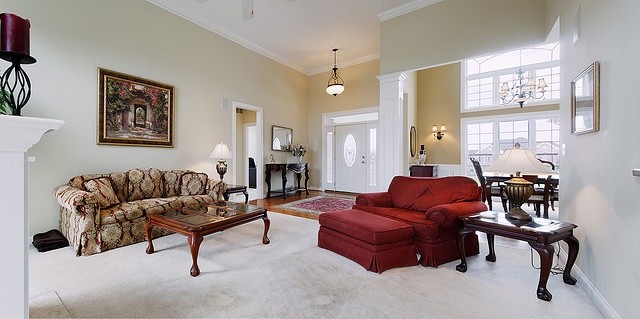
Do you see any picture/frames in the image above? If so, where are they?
[410,125,416,157]
[568,60,601,137]
[95,67,177,149]
[271,124,293,152]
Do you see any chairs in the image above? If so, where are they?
[519,158,555,218]
[469,157,503,206]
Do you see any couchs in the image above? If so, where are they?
[315,174,488,268]
[52,167,228,259]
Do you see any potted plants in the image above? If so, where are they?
[290,144,308,163]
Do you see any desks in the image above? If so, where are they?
[228,184,250,204]
[482,171,559,219]
[264,162,311,200]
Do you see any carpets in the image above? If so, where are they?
[28,205,609,319]
[269,193,359,222]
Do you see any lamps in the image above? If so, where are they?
[495,52,547,112]
[482,141,559,220]
[431,124,440,137]
[440,124,448,136]
[325,48,346,98]
[207,141,229,184]
